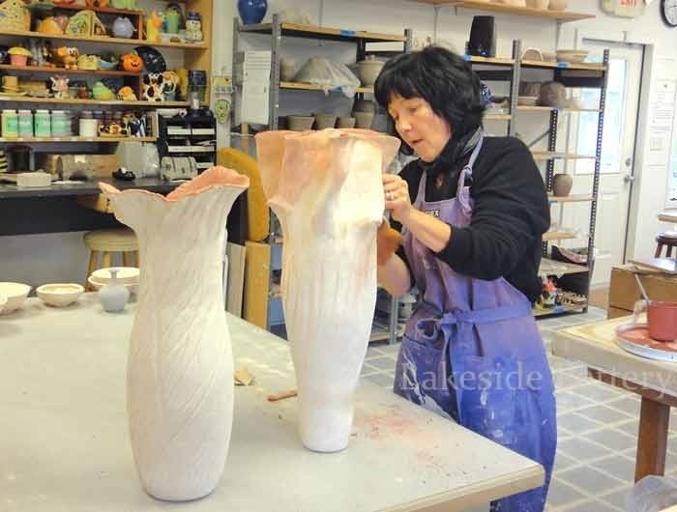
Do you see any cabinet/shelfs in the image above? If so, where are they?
[1,1,215,197]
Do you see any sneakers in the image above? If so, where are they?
[530,278,586,312]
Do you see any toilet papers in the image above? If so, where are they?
[80,118,98,137]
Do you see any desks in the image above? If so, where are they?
[0,287,546,511]
[549,310,677,487]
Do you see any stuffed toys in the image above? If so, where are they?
[49,12,204,138]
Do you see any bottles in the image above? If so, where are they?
[358,53,385,88]
[96,268,131,313]
[352,99,377,129]
[2,106,74,139]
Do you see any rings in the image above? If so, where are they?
[387,191,396,201]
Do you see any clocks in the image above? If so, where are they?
[660,0,677,27]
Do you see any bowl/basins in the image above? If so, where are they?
[87,265,140,297]
[278,110,356,130]
[0,281,84,314]
[556,48,590,62]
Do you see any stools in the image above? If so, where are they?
[83,228,140,293]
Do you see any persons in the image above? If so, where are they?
[372,41,560,512]
